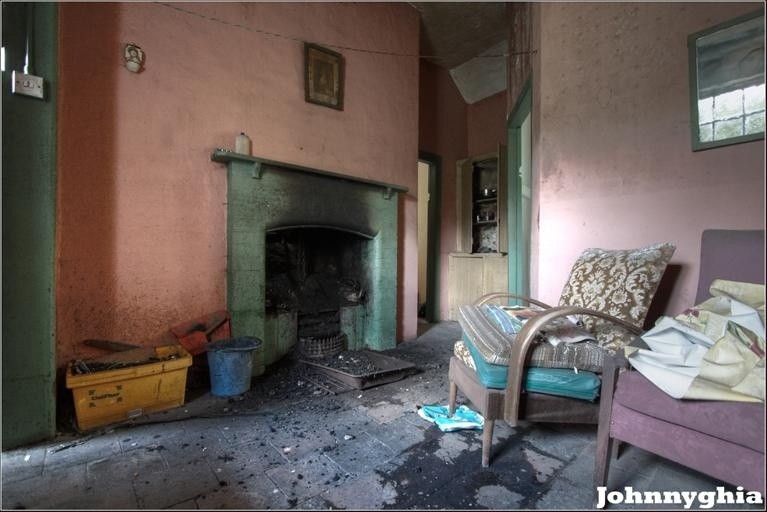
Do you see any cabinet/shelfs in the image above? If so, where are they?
[449,143,508,321]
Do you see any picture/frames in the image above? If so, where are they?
[303,41,344,111]
[687,8,764,152]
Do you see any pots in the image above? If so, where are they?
[480,187,497,198]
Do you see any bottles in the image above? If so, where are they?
[233,130,252,156]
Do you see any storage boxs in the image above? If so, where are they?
[66,344,193,429]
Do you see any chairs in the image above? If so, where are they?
[448,240,677,467]
[593,229,765,500]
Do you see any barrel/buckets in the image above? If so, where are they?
[203,335,262,397]
